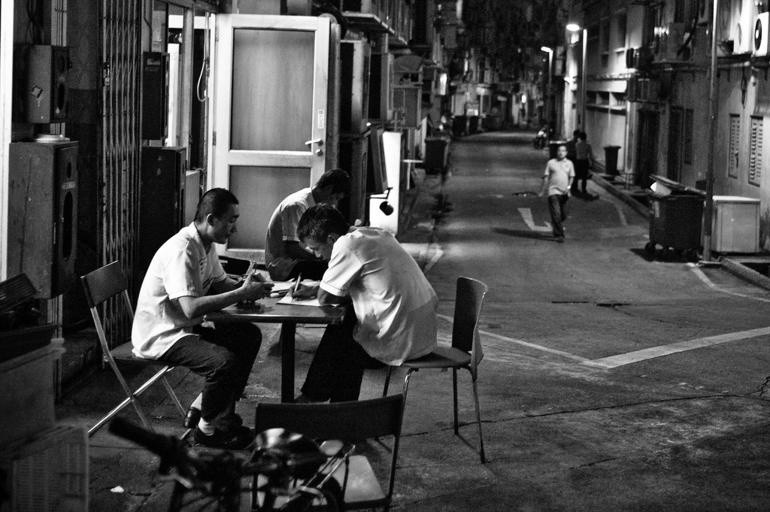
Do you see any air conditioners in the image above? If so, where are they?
[751,11,769,57]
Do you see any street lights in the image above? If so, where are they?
[541,46,554,144]
[566,23,588,134]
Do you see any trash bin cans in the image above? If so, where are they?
[425,137,451,175]
[454,115,501,137]
[549,140,570,160]
[603,146,621,176]
[643,188,707,263]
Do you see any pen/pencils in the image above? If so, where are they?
[292,272,302,299]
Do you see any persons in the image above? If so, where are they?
[573,132,594,195]
[264,168,353,282]
[564,129,581,195]
[285,201,440,409]
[538,144,576,243]
[130,187,275,452]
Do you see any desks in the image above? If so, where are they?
[201,293,350,404]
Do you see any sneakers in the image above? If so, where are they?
[183,407,243,429]
[196,427,254,451]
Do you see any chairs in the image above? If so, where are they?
[376,276,490,512]
[80,260,186,438]
[251,393,406,512]
